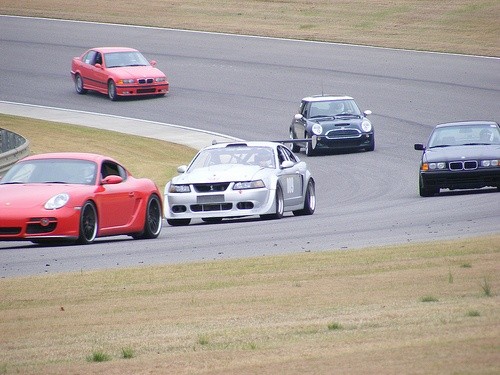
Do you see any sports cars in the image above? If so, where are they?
[0,150,163,245]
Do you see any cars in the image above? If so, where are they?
[289,93,375,157]
[70,47,169,102]
[162,140,317,226]
[414,120,500,197]
